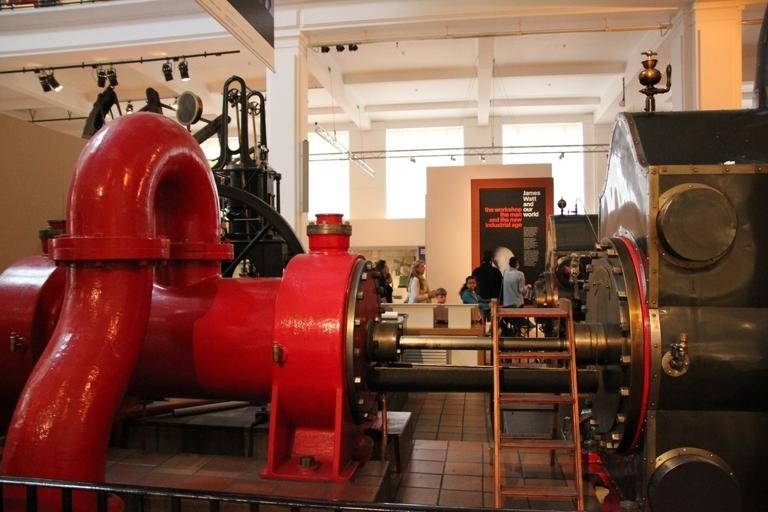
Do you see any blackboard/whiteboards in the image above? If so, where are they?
[471,177,554,308]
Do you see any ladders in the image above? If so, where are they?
[490,297,584,512]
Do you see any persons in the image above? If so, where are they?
[372,257,395,304]
[470,248,503,333]
[434,286,448,305]
[502,255,534,324]
[405,258,436,304]
[458,275,479,304]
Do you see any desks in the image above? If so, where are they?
[380,302,485,363]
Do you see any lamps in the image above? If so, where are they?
[33,54,197,95]
[318,42,358,55]
